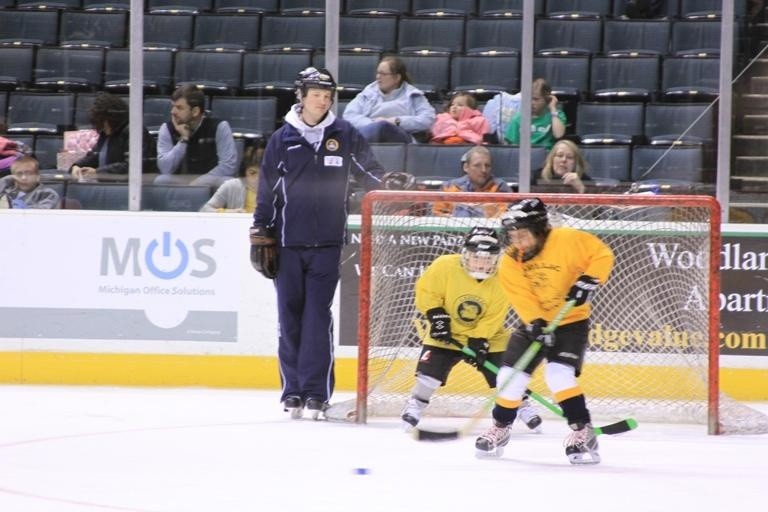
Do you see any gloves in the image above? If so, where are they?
[383,172,416,190]
[565,275,600,306]
[427,308,450,341]
[250,227,278,279]
[526,319,556,349]
[465,337,489,366]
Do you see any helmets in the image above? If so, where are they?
[461,226,498,279]
[295,67,336,99]
[501,198,549,261]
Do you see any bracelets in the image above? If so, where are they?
[550,110,561,116]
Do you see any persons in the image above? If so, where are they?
[342,55,437,143]
[531,140,597,193]
[67,93,154,185]
[248,69,417,409]
[426,146,516,219]
[503,78,568,146]
[200,137,268,214]
[399,226,542,430]
[154,83,240,186]
[427,90,489,144]
[472,196,618,453]
[0,155,59,209]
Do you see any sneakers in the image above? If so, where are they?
[402,397,429,425]
[285,398,322,409]
[565,423,598,455]
[519,401,541,429]
[476,427,510,450]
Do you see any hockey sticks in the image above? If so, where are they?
[413,299,578,441]
[445,339,637,435]
